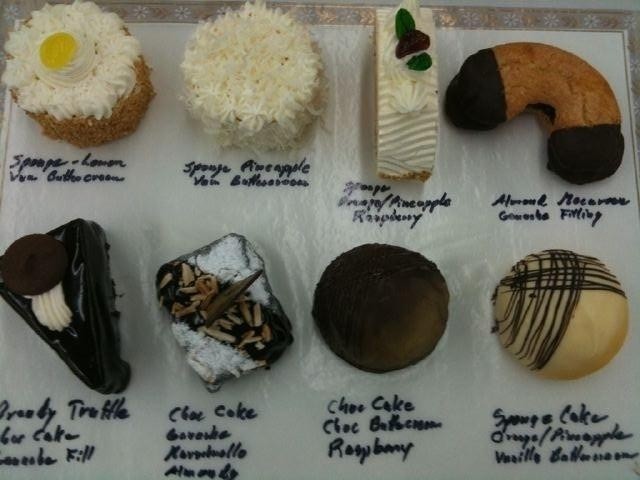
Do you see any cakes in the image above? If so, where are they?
[179,1,331,155]
[2,1,156,148]
[0,218,131,396]
[490,249,630,380]
[310,242,449,374]
[155,232,294,392]
[372,1,439,183]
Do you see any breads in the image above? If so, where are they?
[444,43,622,184]
